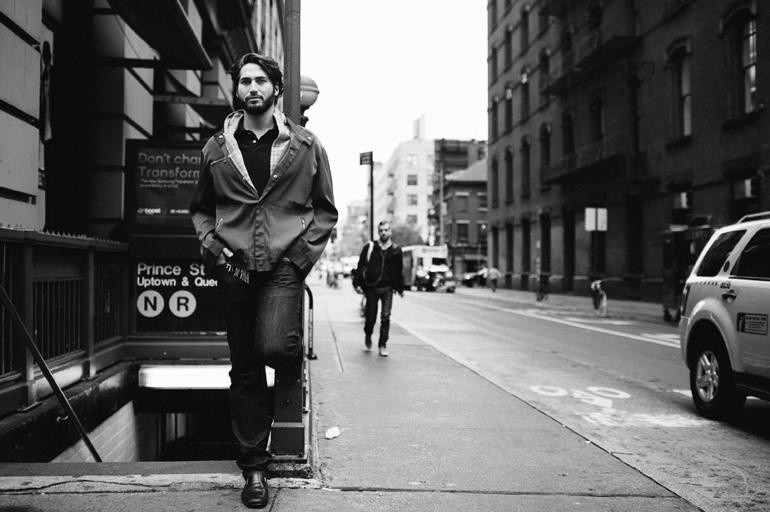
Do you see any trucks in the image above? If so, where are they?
[401,245,456,293]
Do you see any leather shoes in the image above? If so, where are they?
[242,470,268,508]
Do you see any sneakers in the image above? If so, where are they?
[365,343,388,356]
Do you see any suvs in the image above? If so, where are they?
[678,212,770,420]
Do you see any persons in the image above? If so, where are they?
[488,264,502,293]
[353,219,405,357]
[189,52,340,509]
[478,264,487,285]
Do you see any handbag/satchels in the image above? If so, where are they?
[358,262,370,289]
